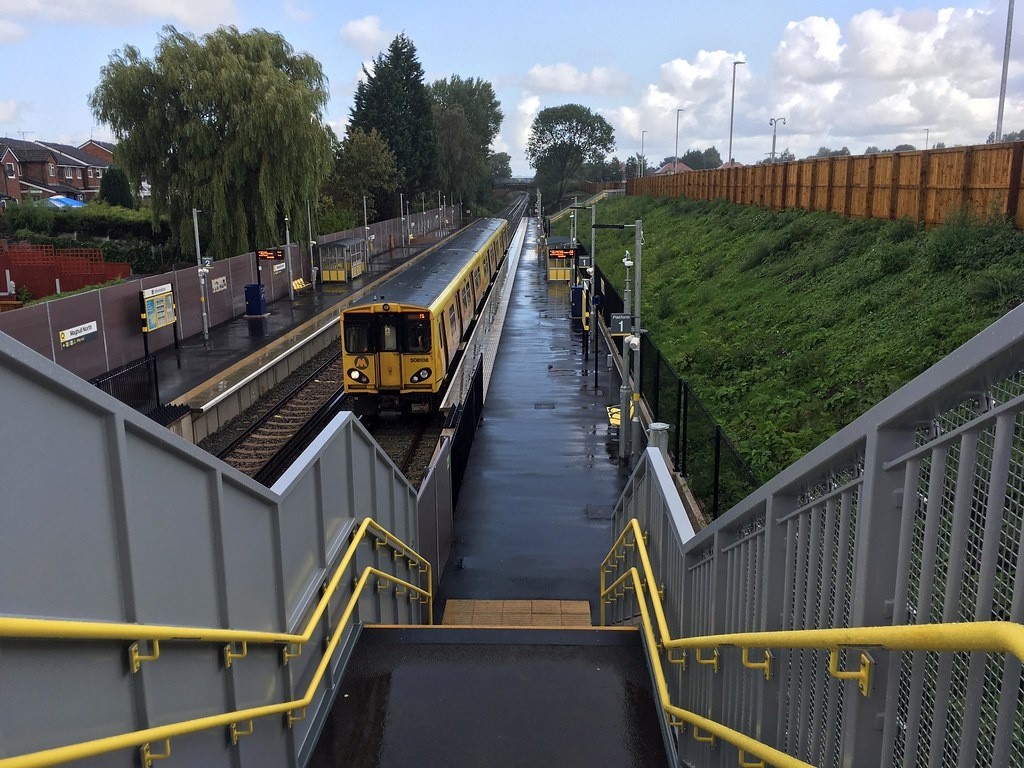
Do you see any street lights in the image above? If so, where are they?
[363,195,379,264]
[193,207,218,341]
[728,61,746,168]
[307,198,323,290]
[923,128,929,150]
[674,108,685,173]
[769,117,787,163]
[641,130,648,177]
[570,205,596,351]
[590,219,642,473]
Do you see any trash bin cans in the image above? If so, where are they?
[571,285,589,317]
[244,284,266,315]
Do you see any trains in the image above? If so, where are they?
[339,217,509,416]
